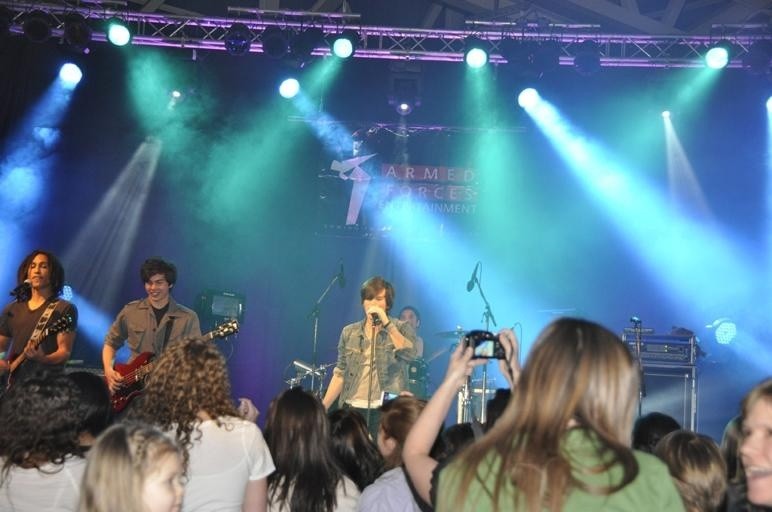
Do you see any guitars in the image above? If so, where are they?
[0,314,73,398]
[104,316,240,414]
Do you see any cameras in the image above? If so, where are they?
[381,392,400,407]
[466,330,505,359]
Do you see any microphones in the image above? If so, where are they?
[340,263,345,286]
[371,313,381,325]
[630,316,642,323]
[10,279,31,295]
[467,261,479,291]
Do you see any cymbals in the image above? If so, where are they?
[434,330,469,338]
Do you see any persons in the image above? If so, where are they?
[321,278,424,442]
[0,250,77,372]
[1,371,112,511]
[359,388,510,512]
[101,258,202,396]
[131,337,275,511]
[632,378,772,511]
[263,387,385,511]
[398,307,420,331]
[402,317,688,512]
[79,423,187,511]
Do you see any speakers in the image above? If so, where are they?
[631,361,697,433]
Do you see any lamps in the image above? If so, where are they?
[1,0,772,116]
